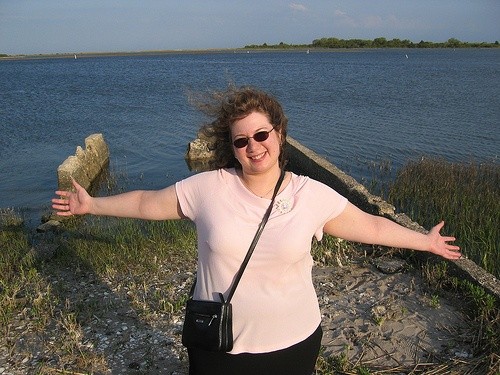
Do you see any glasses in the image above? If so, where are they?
[231,126,276,148]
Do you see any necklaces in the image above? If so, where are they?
[239,173,289,198]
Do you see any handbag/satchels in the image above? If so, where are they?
[182,300,234,352]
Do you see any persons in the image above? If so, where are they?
[52,84,461,375]
